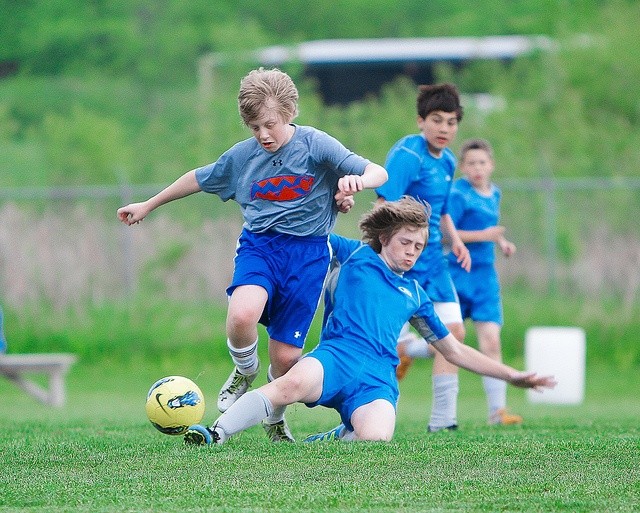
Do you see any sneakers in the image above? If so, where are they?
[396,333,419,381]
[485,407,522,428]
[183,425,222,447]
[262,416,297,443]
[217,355,261,413]
[301,422,346,444]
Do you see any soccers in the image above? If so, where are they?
[146,377,205,435]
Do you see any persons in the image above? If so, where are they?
[116,67,388,444]
[375,84,471,433]
[395,139,521,441]
[184,194,558,447]
